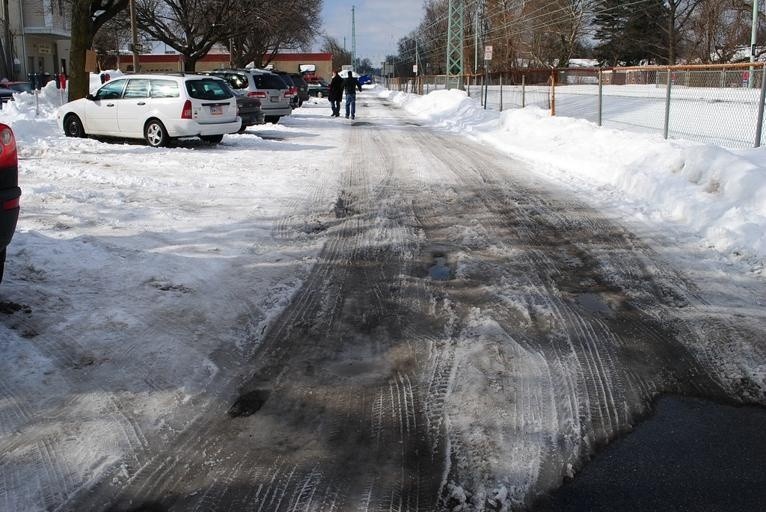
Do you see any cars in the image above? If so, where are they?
[0,123,22,284]
[57,68,330,147]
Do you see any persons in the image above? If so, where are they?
[106,74,111,81]
[342,71,362,120]
[329,69,344,117]
[100,73,105,84]
